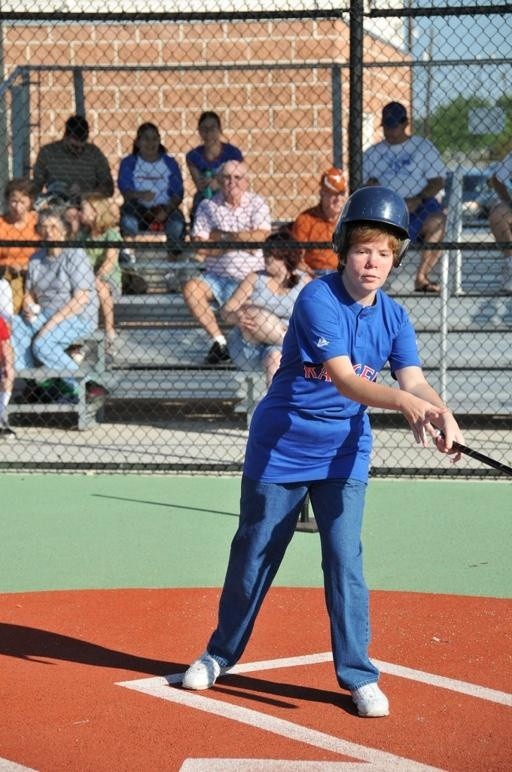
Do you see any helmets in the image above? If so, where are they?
[331,183,410,268]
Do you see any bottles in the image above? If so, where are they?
[203,171,215,200]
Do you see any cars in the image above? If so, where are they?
[438,165,490,220]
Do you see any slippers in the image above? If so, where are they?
[413,281,442,293]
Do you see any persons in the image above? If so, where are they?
[180,186,466,717]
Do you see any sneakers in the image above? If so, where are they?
[182,648,235,690]
[204,340,231,365]
[349,682,392,718]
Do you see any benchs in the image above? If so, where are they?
[1,221,512,425]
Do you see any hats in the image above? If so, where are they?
[378,102,407,128]
[319,168,346,194]
